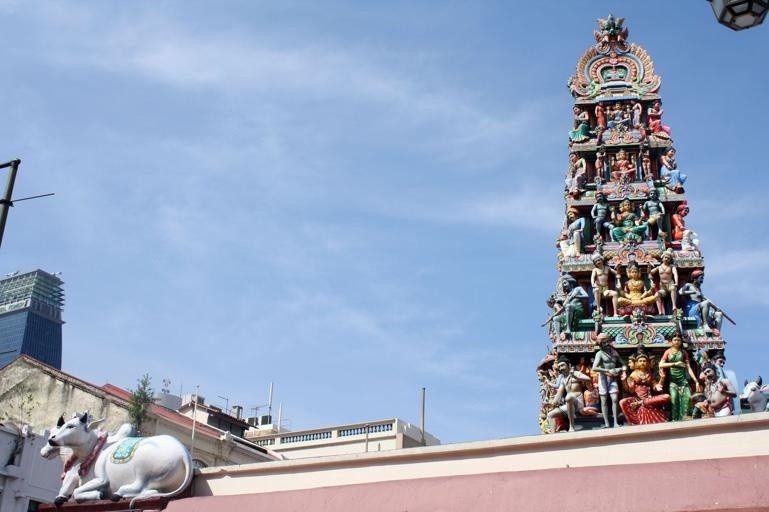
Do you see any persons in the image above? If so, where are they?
[540,103,736,434]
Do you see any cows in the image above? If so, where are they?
[41,411,194,510]
[740,376,769,412]
